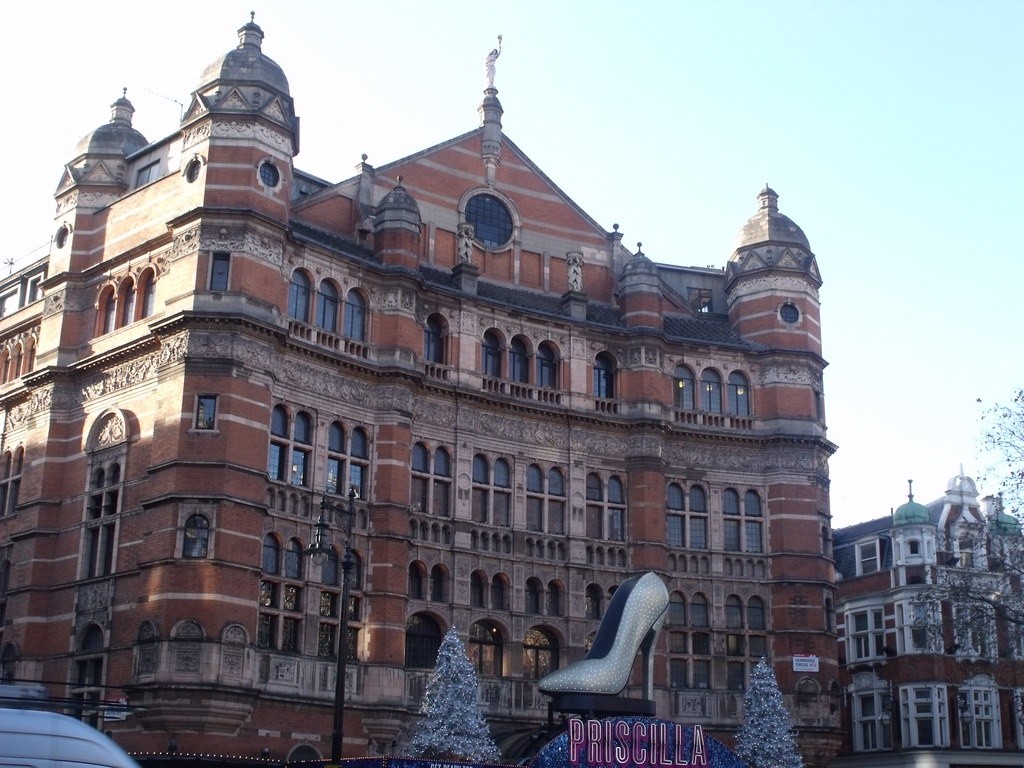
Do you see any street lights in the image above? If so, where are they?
[302,486,355,767]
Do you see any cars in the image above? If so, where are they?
[0,705,139,767]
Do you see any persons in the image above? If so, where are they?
[485,34,502,89]
[564,256,583,291]
[454,227,476,264]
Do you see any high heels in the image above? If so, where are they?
[540,568,672,701]
